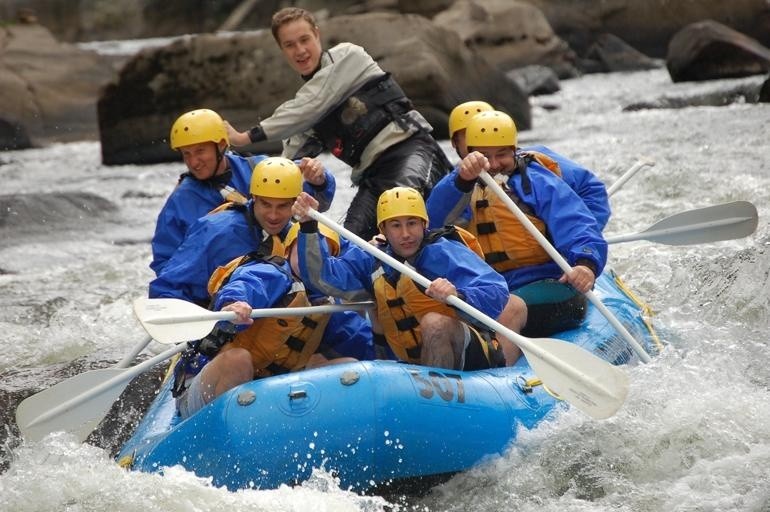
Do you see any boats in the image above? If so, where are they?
[107,268,662,496]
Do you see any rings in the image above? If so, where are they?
[312,166,318,171]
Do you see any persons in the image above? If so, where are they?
[425,109,609,366]
[219,6,455,244]
[150,155,304,350]
[172,216,374,422]
[289,184,511,372]
[448,100,613,234]
[147,109,336,277]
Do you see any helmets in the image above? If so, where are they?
[448,100,494,148]
[282,218,341,264]
[465,110,518,156]
[249,156,303,198]
[169,108,230,152]
[376,186,430,234]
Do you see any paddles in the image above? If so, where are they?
[134,298,377,345]
[294,205,627,420]
[16,342,188,442]
[604,201,758,246]
